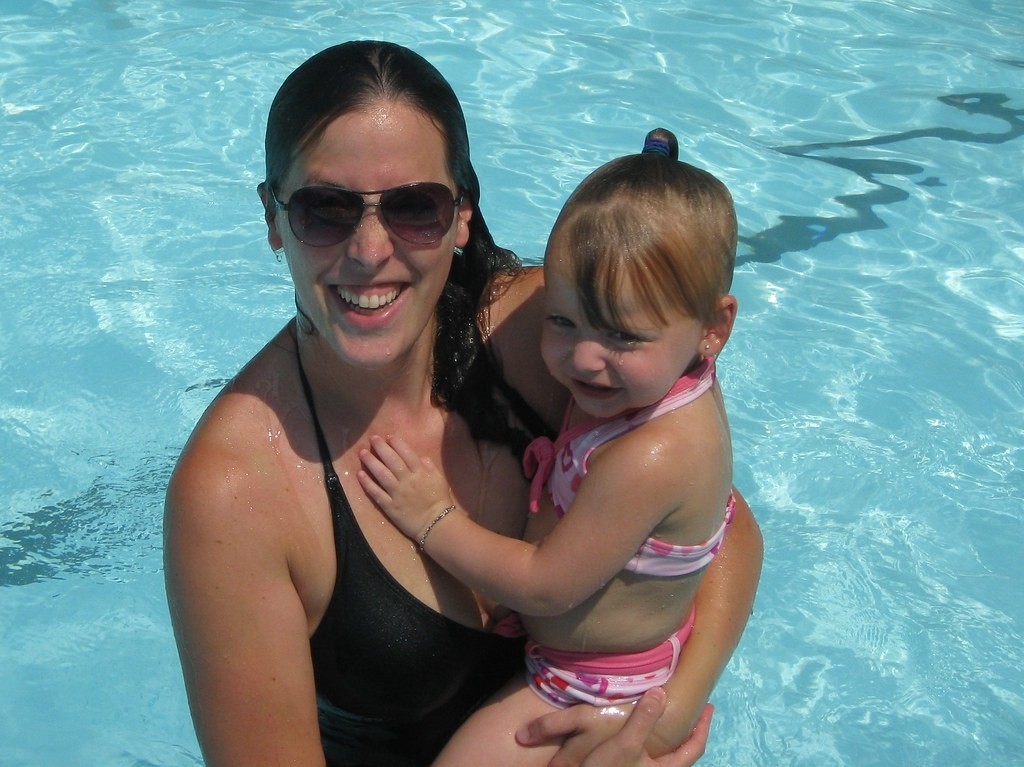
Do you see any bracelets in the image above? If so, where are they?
[421,505,456,553]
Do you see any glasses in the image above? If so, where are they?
[269,182,466,248]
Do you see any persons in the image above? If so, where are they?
[358,128,738,767]
[164,42,763,767]
